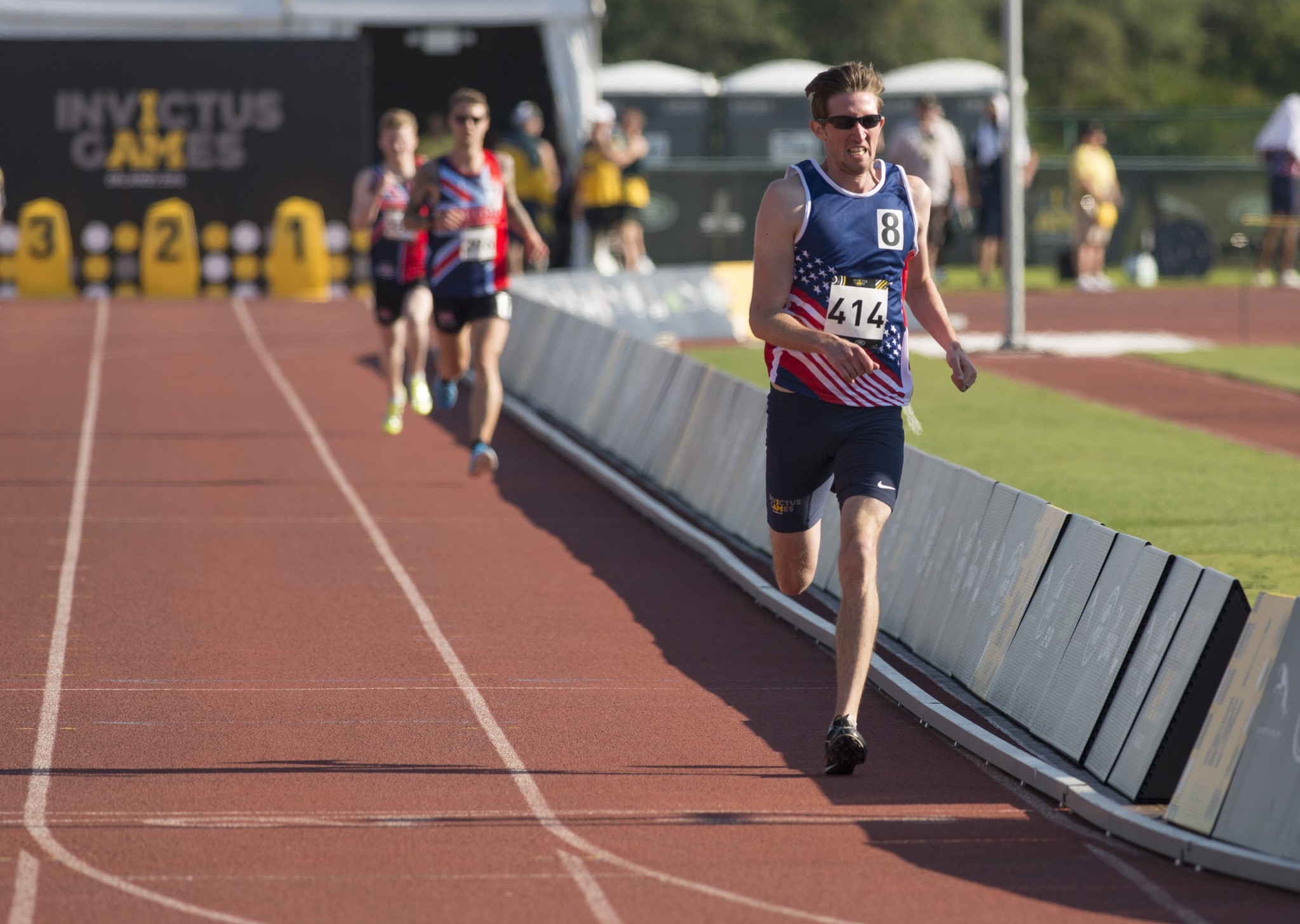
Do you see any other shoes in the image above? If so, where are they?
[1283,270,1300,289]
[1094,276,1113,293]
[383,385,406,435]
[436,373,458,410]
[470,443,497,482]
[408,373,433,416]
[1252,270,1275,288]
[1076,276,1103,292]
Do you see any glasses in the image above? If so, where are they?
[451,116,485,123]
[816,114,882,129]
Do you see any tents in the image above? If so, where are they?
[594,54,1013,165]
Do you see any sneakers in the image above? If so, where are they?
[825,715,866,776]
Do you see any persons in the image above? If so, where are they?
[967,90,1040,285]
[749,60,978,775]
[568,99,650,271]
[1066,120,1124,294]
[1250,93,1300,288]
[886,95,969,278]
[351,109,434,435]
[404,88,551,478]
[494,100,560,272]
